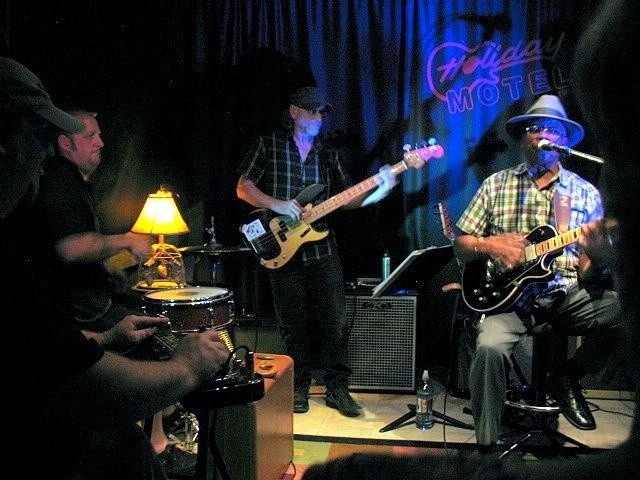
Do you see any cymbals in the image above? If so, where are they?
[177,245,250,256]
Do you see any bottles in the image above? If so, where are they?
[380,249,390,285]
[416,371,435,432]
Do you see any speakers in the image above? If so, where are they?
[345,294,420,393]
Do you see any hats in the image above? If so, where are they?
[505,95,584,149]
[0,57,86,134]
[291,86,334,112]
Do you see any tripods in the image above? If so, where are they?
[381,276,474,431]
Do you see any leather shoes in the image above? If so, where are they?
[294,382,309,412]
[549,377,596,430]
[157,444,199,476]
[325,388,362,417]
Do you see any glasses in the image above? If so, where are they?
[524,124,562,133]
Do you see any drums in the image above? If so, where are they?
[144,286,234,332]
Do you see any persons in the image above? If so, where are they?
[455,92,626,459]
[46,105,203,472]
[0,53,239,479]
[232,85,398,418]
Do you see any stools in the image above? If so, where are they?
[473,316,589,479]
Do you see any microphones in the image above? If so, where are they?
[537,138,570,156]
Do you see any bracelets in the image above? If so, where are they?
[474,237,485,253]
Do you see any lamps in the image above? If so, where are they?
[129,184,190,289]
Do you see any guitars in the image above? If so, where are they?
[237,138,445,269]
[461,219,620,314]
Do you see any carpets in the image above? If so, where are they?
[294,388,639,452]
